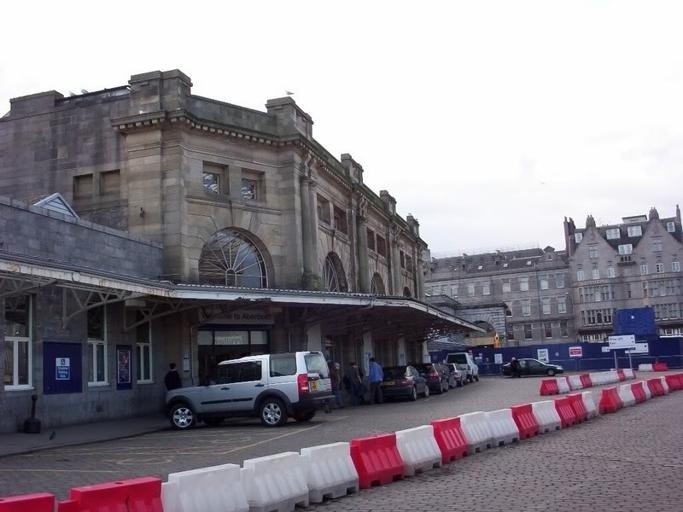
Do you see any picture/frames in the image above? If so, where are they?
[116,344,134,389]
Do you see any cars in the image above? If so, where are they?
[409,362,464,394]
[380,365,430,401]
[502,357,564,376]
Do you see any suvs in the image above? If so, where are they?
[446,353,479,383]
[165,349,336,431]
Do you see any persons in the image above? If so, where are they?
[328,360,345,408]
[511,357,521,377]
[164,363,182,390]
[346,361,367,406]
[367,357,384,403]
